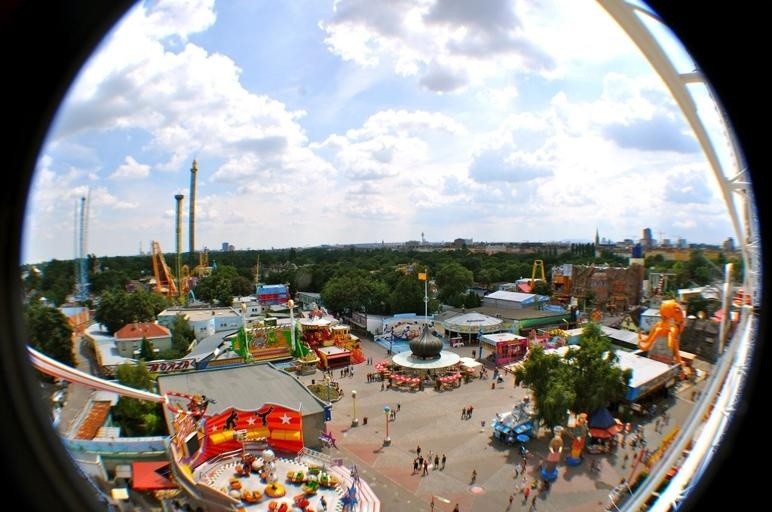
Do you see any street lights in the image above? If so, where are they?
[327,377,330,401]
[352,390,357,421]
[384,407,390,438]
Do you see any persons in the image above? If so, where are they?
[397,400,401,410]
[509,442,538,506]
[471,470,476,482]
[413,445,446,476]
[697,392,703,400]
[691,390,696,400]
[461,405,473,419]
[590,413,670,473]
[367,357,370,365]
[370,357,373,364]
[367,372,375,383]
[479,365,512,382]
[452,503,460,512]
[255,406,273,425]
[340,365,354,378]
[320,496,327,511]
[222,409,239,430]
